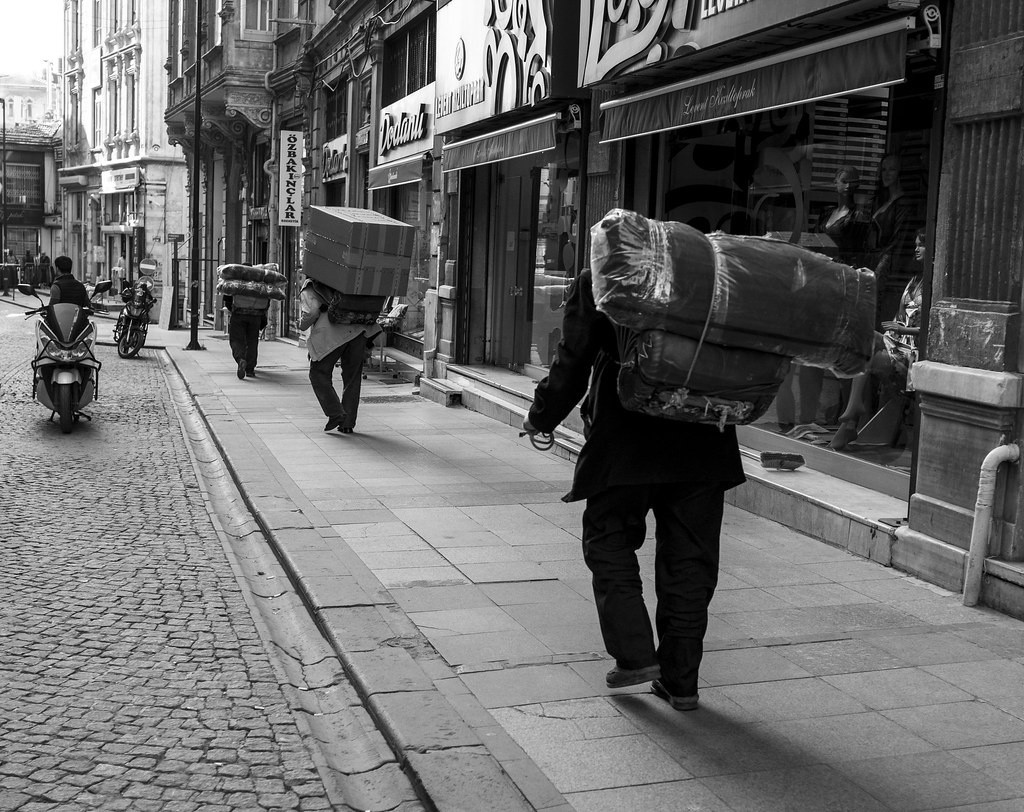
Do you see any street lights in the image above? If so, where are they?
[0,98,11,297]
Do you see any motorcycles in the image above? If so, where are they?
[15,280,113,435]
[113,269,158,359]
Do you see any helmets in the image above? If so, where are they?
[122,287,133,304]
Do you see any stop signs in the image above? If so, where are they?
[139,259,156,275]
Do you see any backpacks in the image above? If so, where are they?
[616,324,792,434]
[329,289,387,327]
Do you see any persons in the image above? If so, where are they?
[825,227,926,450]
[223,262,268,377]
[7,249,19,265]
[117,251,125,268]
[814,149,916,419]
[40,252,50,264]
[22,249,34,265]
[298,275,382,433]
[521,209,789,712]
[48,256,92,324]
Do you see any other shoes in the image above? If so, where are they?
[245,369,256,377]
[606,662,659,688]
[338,426,354,434]
[323,415,347,431]
[238,360,246,379]
[648,679,699,710]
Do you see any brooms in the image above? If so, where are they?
[739,448,806,471]
